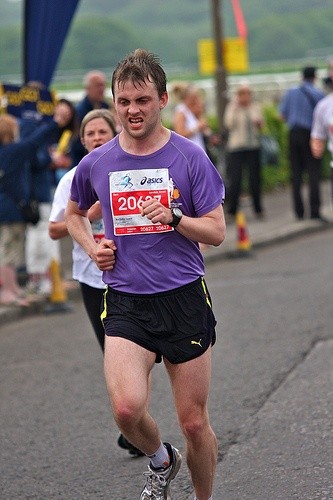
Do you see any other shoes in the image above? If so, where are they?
[117,432,145,455]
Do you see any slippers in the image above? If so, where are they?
[0,293,41,308]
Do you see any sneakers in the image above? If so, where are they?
[139,442,182,500]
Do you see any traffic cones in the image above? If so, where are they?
[234,211,255,257]
[42,258,74,314]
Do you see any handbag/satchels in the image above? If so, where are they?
[21,197,40,225]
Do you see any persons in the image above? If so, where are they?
[169,83,212,251]
[309,58,333,203]
[47,108,148,458]
[321,77,333,95]
[63,47,227,499]
[188,95,221,168]
[0,68,123,311]
[221,86,269,225]
[276,65,328,221]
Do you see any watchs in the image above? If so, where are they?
[167,206,182,228]
